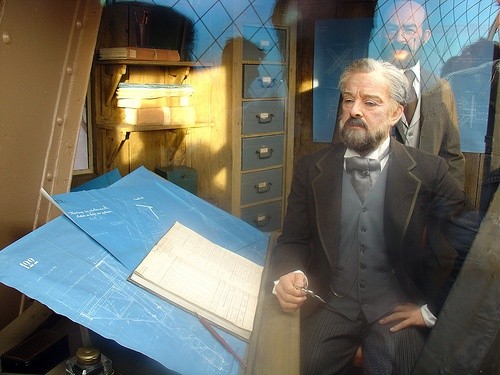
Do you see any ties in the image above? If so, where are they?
[400,69,419,126]
[345,145,391,205]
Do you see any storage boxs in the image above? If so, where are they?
[155,165,198,196]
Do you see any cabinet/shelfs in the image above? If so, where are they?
[186,4,297,234]
[94,48,214,178]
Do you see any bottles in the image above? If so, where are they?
[72,347,105,375]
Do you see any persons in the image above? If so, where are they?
[267,59,480,375]
[382,2,468,206]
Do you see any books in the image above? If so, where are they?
[99,46,182,61]
[116,82,196,124]
[126,221,265,345]
